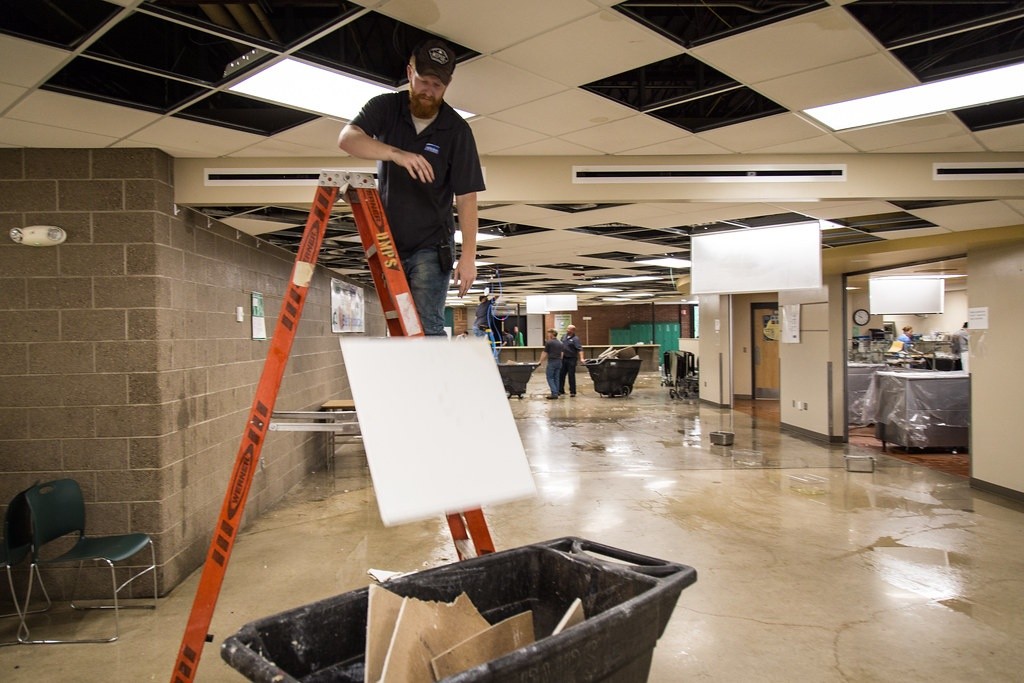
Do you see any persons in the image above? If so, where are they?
[514,326,525,346]
[949,321,968,353]
[473,296,499,336]
[457,331,468,341]
[559,324,586,397]
[896,325,924,355]
[501,328,513,346]
[337,40,487,335]
[537,329,565,399]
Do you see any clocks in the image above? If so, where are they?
[853,307,871,326]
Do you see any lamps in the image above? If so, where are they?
[9,225,69,247]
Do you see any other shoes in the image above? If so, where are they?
[545,392,576,400]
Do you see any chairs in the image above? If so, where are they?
[16,478,158,643]
[0,478,51,646]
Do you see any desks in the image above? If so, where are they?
[321,399,369,471]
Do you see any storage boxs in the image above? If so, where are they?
[842,454,876,473]
[709,430,736,445]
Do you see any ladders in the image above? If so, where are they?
[169,171,494,683]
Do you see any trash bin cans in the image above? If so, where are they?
[581,358,643,397]
[497,362,541,399]
[222,537,697,683]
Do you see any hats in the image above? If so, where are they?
[479,295,488,302]
[411,38,456,87]
